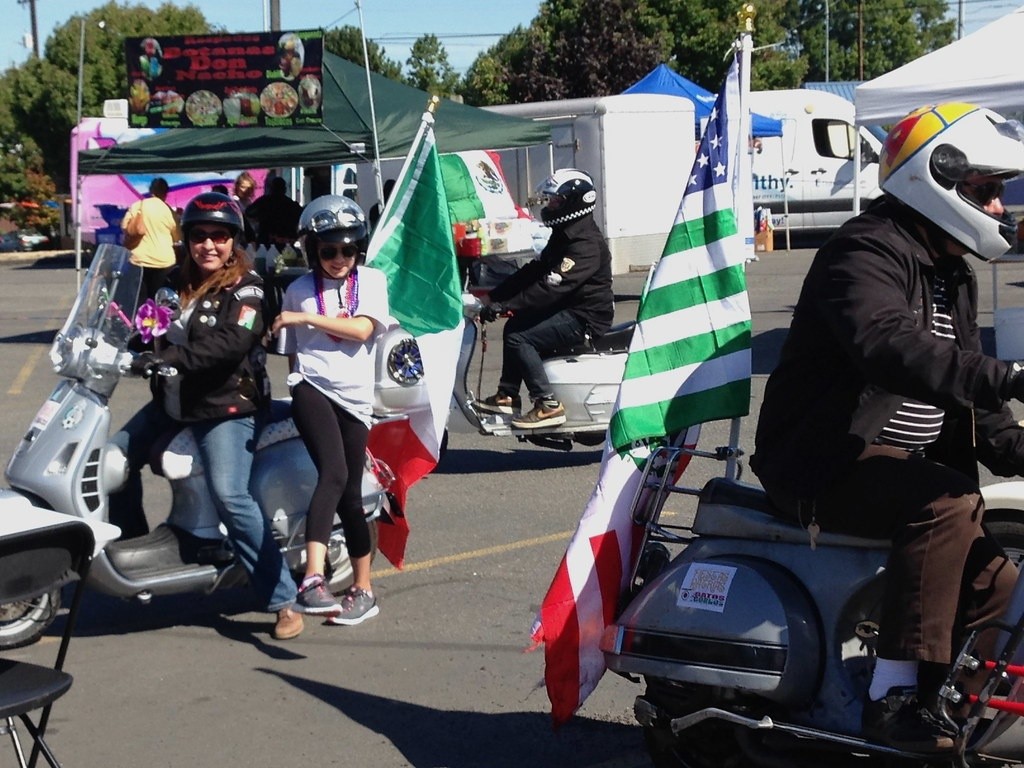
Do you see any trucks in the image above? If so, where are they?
[69,96,694,297]
[696,91,889,230]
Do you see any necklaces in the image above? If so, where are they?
[315,266,361,339]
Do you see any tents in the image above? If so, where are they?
[69,116,263,249]
[72,40,559,297]
[616,59,791,254]
[852,0,1024,217]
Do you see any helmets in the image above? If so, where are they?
[298,195,369,270]
[180,192,245,251]
[540,167,598,228]
[879,103,1023,263]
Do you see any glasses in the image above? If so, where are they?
[965,181,1003,204]
[188,229,233,243]
[319,243,359,260]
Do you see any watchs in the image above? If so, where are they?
[1011,361,1024,379]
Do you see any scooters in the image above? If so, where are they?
[439,254,636,460]
[599,446,1024,768]
[0,244,465,648]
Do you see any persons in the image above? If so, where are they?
[117,177,185,300]
[261,191,390,630]
[230,173,256,213]
[212,185,229,195]
[106,189,306,642]
[473,165,616,429]
[742,92,1024,756]
[245,175,302,268]
[369,178,398,231]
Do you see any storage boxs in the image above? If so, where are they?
[754,209,773,251]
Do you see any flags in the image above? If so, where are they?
[364,112,466,566]
[527,45,754,731]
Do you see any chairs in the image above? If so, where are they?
[542,261,659,359]
[0,521,96,768]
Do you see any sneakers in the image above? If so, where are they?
[860,695,955,752]
[471,393,514,415]
[295,573,343,615]
[328,584,379,626]
[511,401,565,430]
[275,606,305,639]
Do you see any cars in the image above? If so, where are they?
[0,229,50,251]
